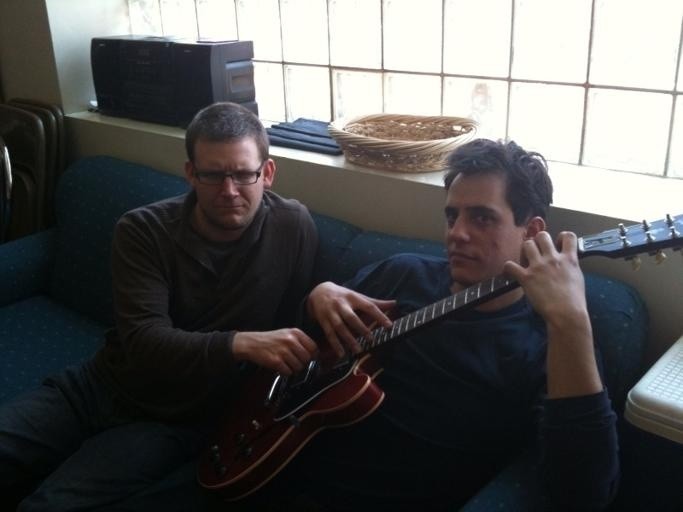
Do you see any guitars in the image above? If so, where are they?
[195,215,683,499]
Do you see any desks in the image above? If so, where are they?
[623,334,682,444]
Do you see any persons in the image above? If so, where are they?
[0,102,318,511]
[109,138,619,510]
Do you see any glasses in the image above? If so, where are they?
[191,164,264,186]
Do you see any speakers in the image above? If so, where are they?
[170,40,258,131]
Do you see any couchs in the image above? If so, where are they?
[1,156,650,511]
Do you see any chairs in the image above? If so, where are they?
[0,99,66,244]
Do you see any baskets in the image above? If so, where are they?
[327,113,481,173]
